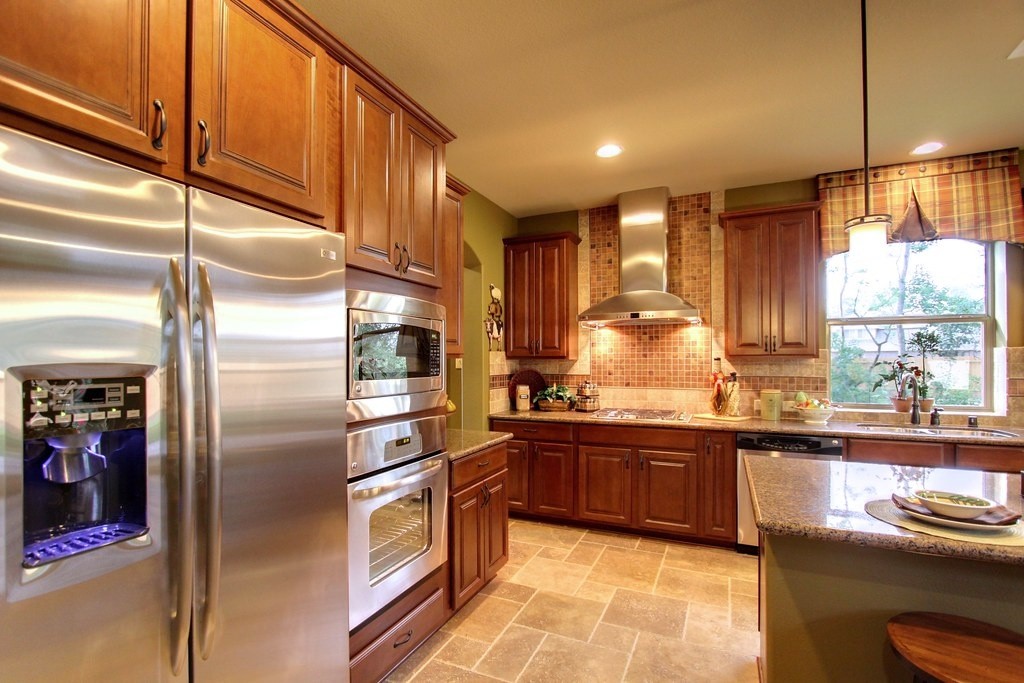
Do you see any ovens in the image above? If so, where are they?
[346,414,448,631]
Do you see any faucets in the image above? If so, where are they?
[898,375,921,424]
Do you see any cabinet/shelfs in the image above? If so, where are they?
[341,37,459,290]
[436,170,473,360]
[718,200,827,359]
[949,444,1024,474]
[699,430,737,549]
[447,441,509,618]
[845,438,949,469]
[575,423,700,546]
[0,0,341,234]
[502,231,583,363]
[491,419,576,527]
[346,560,452,683]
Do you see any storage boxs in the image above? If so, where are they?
[760,389,782,421]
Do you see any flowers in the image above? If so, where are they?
[871,353,922,398]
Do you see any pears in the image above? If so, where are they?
[795,391,808,402]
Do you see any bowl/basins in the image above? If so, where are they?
[911,489,997,520]
[793,404,841,424]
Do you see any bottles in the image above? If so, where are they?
[516,385,530,411]
[760,388,782,421]
[575,384,599,409]
[710,358,740,416]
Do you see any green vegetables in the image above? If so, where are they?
[533,385,577,404]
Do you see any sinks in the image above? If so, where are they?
[928,425,1018,438]
[854,424,927,435]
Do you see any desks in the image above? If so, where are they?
[742,454,1024,683]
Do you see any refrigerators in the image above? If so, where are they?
[0,126,351,683]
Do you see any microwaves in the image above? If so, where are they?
[346,288,447,425]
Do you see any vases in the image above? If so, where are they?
[891,396,914,412]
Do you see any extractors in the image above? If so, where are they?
[575,185,702,328]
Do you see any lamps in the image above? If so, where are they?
[843,0,892,275]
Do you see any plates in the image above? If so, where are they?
[903,509,1020,531]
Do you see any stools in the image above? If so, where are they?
[886,611,1024,683]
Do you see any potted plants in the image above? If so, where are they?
[904,330,942,412]
[532,382,576,412]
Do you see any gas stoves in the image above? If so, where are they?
[590,406,692,423]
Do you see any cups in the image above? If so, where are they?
[968,416,977,426]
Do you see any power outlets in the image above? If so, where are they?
[786,401,795,412]
[754,400,761,410]
[782,401,786,412]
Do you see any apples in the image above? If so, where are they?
[797,399,831,409]
